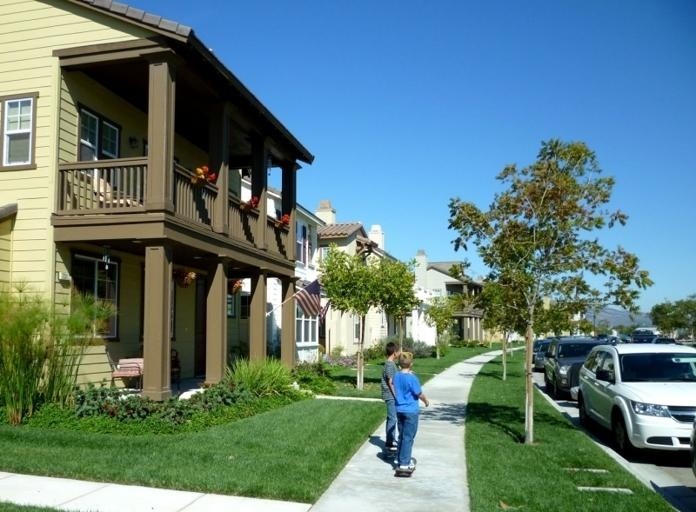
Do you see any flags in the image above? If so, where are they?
[293,279,324,326]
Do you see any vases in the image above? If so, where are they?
[228,286,237,295]
[178,276,188,288]
[190,174,206,187]
[274,220,283,228]
[239,202,249,211]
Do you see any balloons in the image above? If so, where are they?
[247,196,260,210]
[187,164,217,184]
[238,200,250,213]
[272,219,283,228]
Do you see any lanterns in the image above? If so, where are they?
[227,277,241,295]
[174,266,197,290]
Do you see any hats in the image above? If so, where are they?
[399,351,414,360]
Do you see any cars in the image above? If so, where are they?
[532,327,696,478]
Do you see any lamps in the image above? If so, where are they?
[102,246,112,270]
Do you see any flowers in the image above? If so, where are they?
[248,196,260,208]
[186,271,197,285]
[232,279,241,288]
[195,166,216,181]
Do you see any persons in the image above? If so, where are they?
[390,351,430,470]
[379,341,401,454]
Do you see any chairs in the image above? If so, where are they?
[112,357,144,393]
